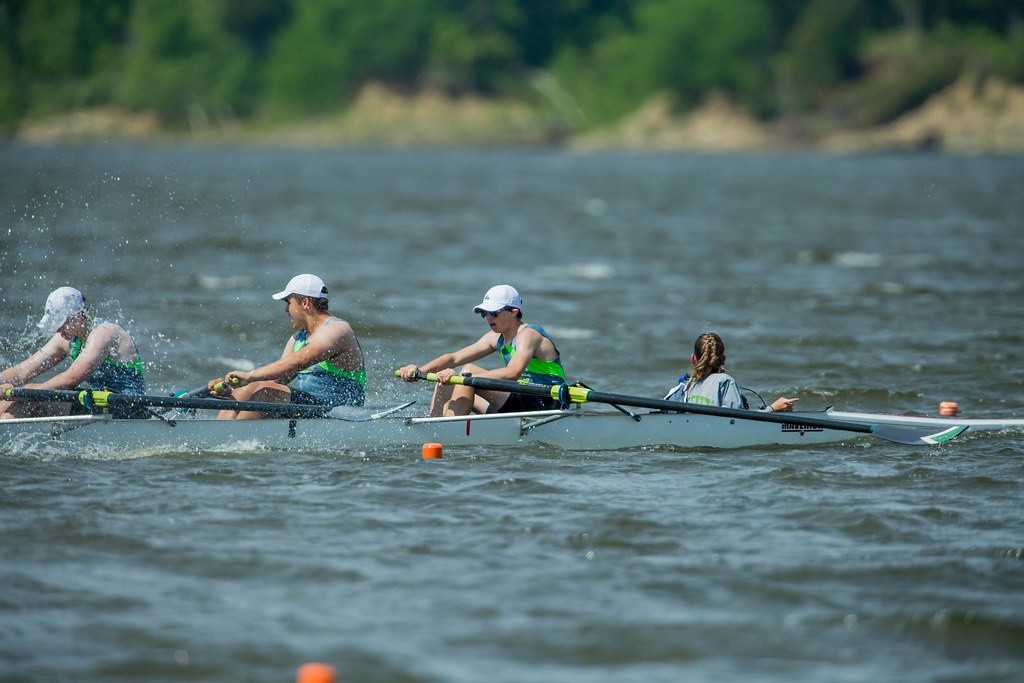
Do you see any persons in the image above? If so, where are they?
[208,273,366,421]
[684,333,800,412]
[401,284,570,418]
[0,286,150,419]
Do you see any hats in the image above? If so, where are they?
[473,285,522,314]
[36,287,85,332]
[272,273,329,301]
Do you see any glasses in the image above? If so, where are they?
[481,309,506,318]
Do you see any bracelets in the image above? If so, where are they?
[770,405,775,412]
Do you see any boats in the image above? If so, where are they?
[0,414,1024,459]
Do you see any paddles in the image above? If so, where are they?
[150,372,243,414]
[0,388,418,424]
[396,368,971,448]
